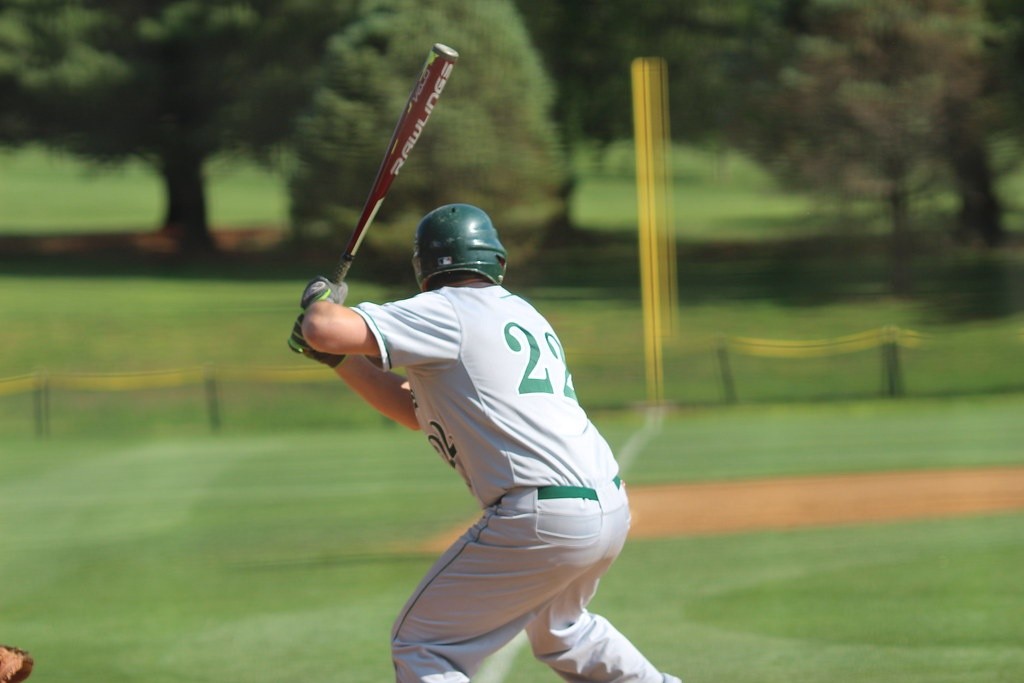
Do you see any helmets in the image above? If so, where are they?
[412,203,508,292]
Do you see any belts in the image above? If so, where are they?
[495,475,621,501]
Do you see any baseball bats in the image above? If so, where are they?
[287,40,458,354]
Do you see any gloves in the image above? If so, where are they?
[300,277,348,306]
[287,313,349,370]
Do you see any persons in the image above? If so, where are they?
[286,204,683,682]
